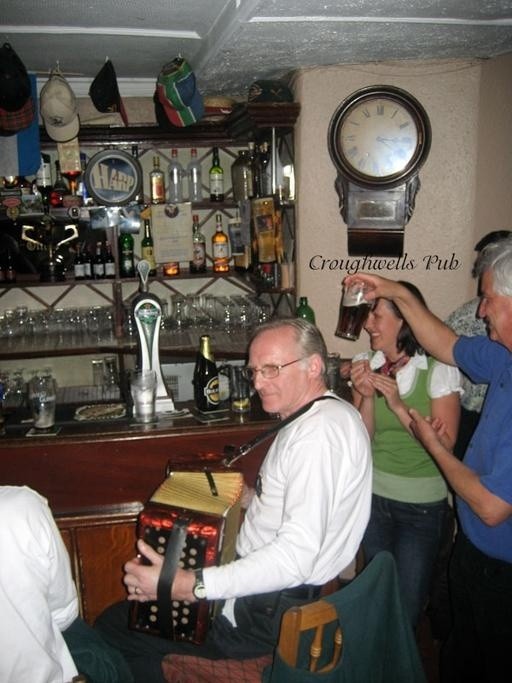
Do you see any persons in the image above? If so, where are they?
[349,280,461,629]
[0,484,131,682]
[443,229,511,463]
[91,320,373,682]
[340,233,511,682]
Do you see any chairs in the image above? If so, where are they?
[163,543,398,683]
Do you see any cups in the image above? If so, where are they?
[276,263,296,289]
[91,356,157,422]
[230,368,250,413]
[336,278,375,342]
[1,293,271,351]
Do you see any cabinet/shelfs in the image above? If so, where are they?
[0,402,285,631]
[0,100,299,435]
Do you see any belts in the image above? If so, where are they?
[234,554,320,599]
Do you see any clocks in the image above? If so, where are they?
[327,83,431,257]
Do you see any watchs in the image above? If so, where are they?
[192,567,207,600]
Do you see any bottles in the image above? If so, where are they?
[296,296,315,325]
[194,334,221,409]
[2,365,59,430]
[0,142,273,284]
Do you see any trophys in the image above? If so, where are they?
[126,292,175,415]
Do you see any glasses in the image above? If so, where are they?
[239,360,302,380]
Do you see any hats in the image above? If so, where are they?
[0,42,36,132]
[156,57,206,128]
[38,68,80,144]
[244,77,290,103]
[87,57,129,129]
